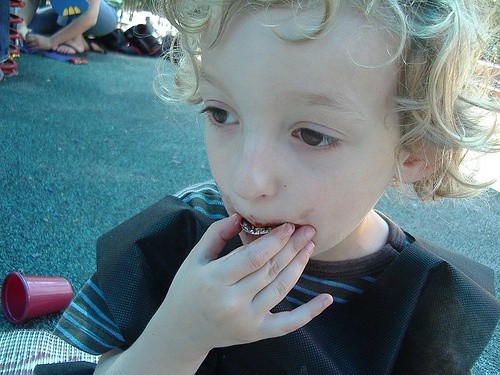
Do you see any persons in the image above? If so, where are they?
[15,0,124,54]
[51,0,500,375]
[145,17,157,34]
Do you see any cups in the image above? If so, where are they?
[2,273,73,321]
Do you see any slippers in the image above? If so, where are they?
[86,39,107,54]
[55,42,87,56]
